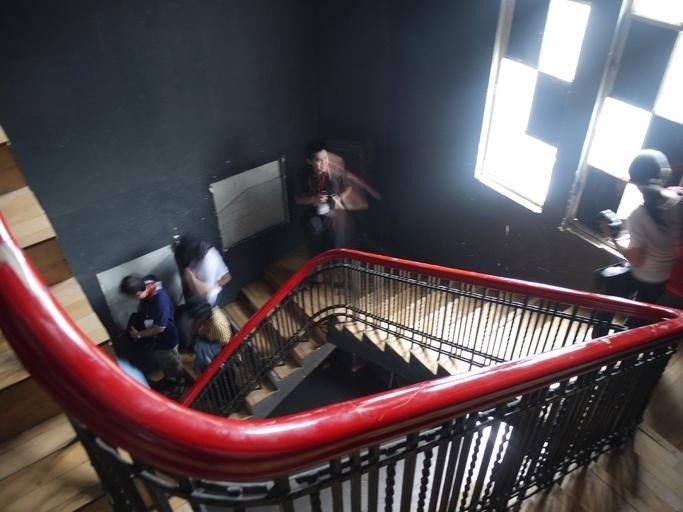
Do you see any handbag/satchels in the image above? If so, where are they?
[117,312,159,353]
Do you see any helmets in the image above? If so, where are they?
[628,148,673,186]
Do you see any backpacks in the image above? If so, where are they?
[668,243,683,297]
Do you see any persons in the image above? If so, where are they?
[293,142,352,283]
[120,233,239,402]
[592,155,683,340]
